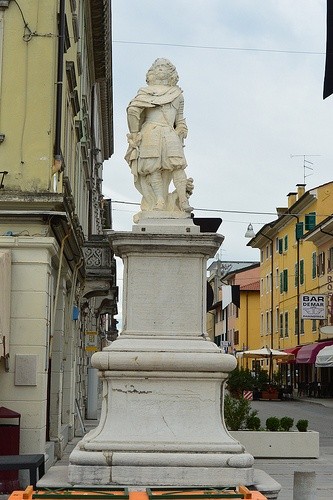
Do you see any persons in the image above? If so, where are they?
[128,57,195,213]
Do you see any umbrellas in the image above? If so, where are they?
[243,345,295,385]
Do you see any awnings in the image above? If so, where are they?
[296,341,333,364]
[315,345,333,367]
[276,346,302,365]
